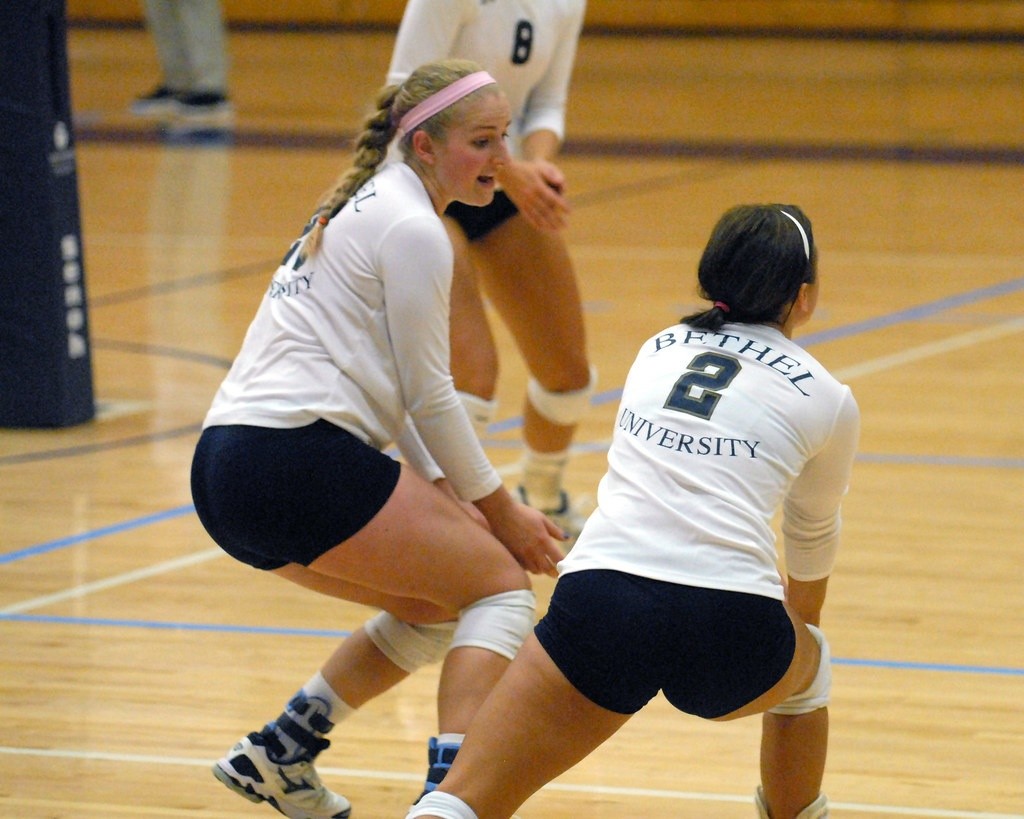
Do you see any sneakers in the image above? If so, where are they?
[212,731,351,818]
[510,486,584,534]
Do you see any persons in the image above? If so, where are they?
[405,202,860,819]
[385,0,599,538]
[129,0,230,115]
[190,56,568,819]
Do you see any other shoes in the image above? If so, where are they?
[132,87,232,123]
